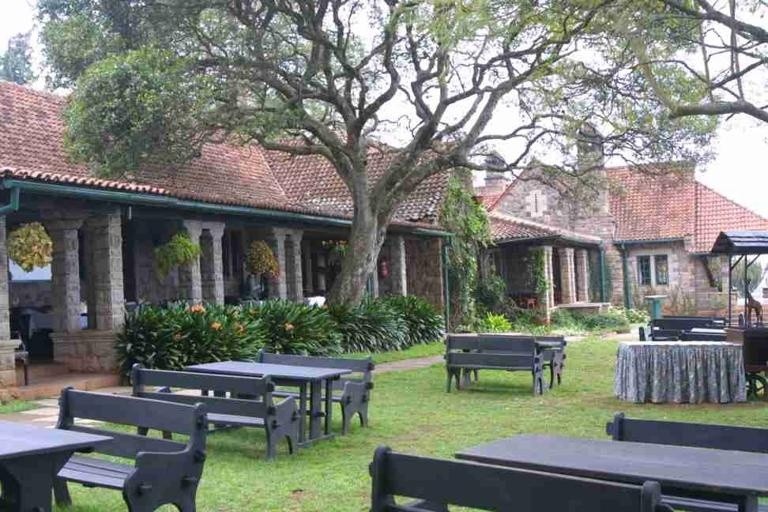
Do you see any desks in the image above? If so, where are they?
[456,433,768,512]
[0,419,114,511]
[537,340,560,386]
[186,359,353,447]
[613,341,747,404]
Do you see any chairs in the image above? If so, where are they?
[11,331,29,387]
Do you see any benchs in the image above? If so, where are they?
[132,363,301,460]
[536,336,566,388]
[445,335,545,397]
[648,316,727,341]
[370,443,674,511]
[607,409,768,451]
[254,348,375,436]
[53,385,208,511]
